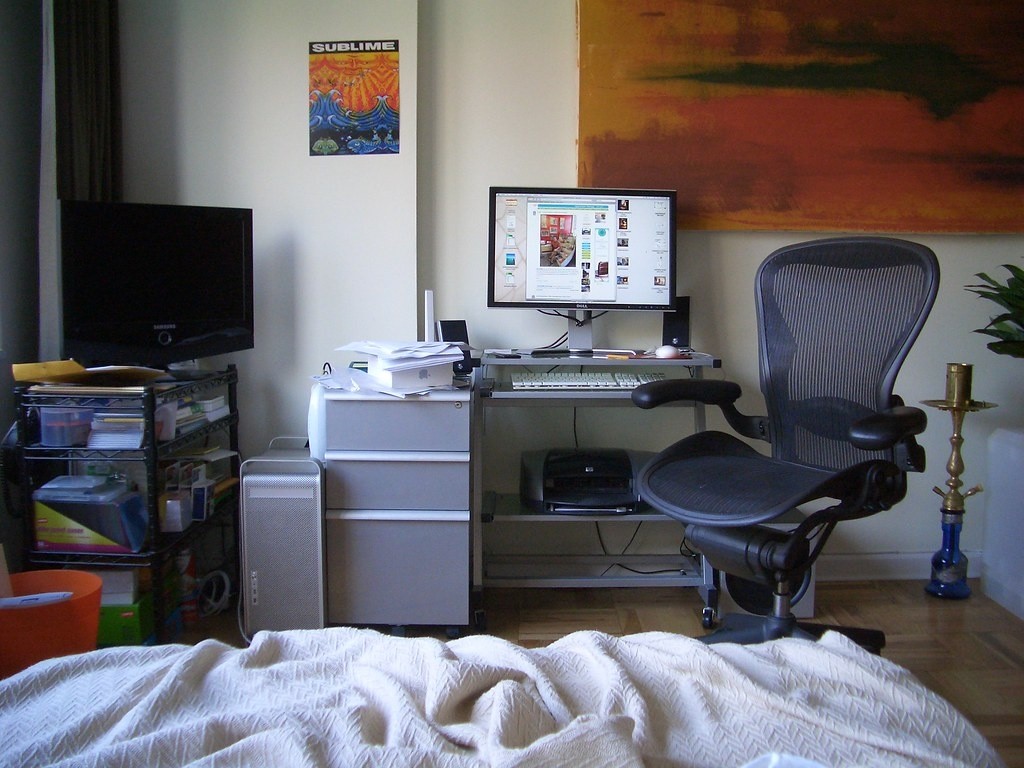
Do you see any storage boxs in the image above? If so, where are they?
[159,490,192,531]
[197,395,224,412]
[38,407,92,448]
[95,568,140,605]
[97,596,152,645]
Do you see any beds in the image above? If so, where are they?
[0,626,998,768]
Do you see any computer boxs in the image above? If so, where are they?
[241,435,327,649]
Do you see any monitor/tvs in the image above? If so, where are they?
[488,187,676,356]
[57,200,254,369]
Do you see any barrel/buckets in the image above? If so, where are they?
[0,571,103,680]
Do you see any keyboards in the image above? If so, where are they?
[510,372,667,390]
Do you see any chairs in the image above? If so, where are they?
[630,235,939,656]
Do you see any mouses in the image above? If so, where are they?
[655,345,680,358]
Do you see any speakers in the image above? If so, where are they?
[662,296,690,352]
[436,319,473,375]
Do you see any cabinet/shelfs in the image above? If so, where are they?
[323,392,471,640]
[12,365,241,644]
[474,353,721,630]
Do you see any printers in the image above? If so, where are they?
[520,447,662,516]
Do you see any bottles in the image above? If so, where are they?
[177,542,199,621]
